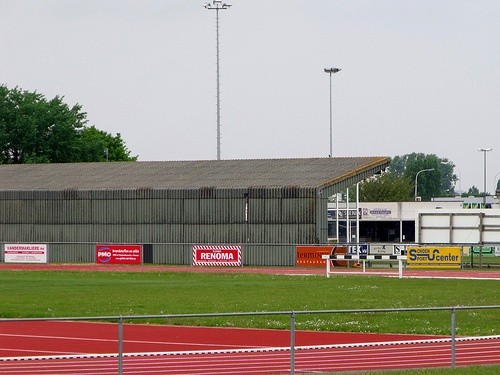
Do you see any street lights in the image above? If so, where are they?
[204,0,232,160]
[414,168,434,202]
[324,68,341,158]
[478,149,493,196]
